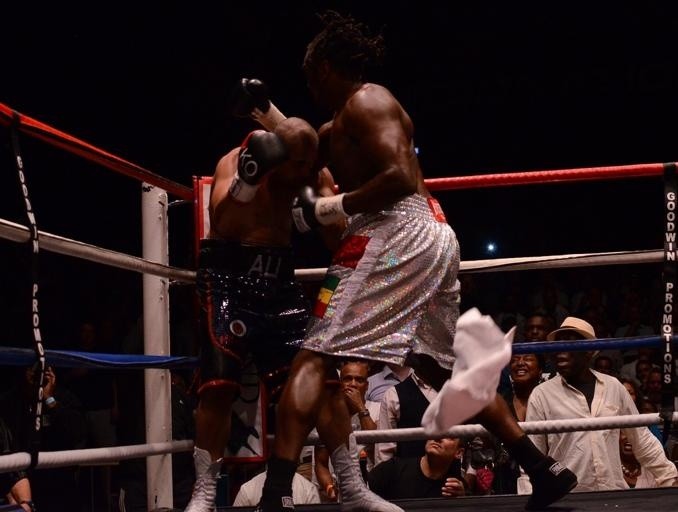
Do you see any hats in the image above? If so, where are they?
[547,317,600,358]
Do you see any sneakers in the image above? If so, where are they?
[513,456,578,509]
[258,487,298,512]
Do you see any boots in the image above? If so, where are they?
[182,446,225,512]
[331,432,405,512]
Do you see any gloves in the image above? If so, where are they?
[292,185,350,233]
[224,77,287,129]
[228,130,286,204]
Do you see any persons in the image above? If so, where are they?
[2,322,118,512]
[184,278,677,511]
[180,115,405,512]
[223,11,578,512]
